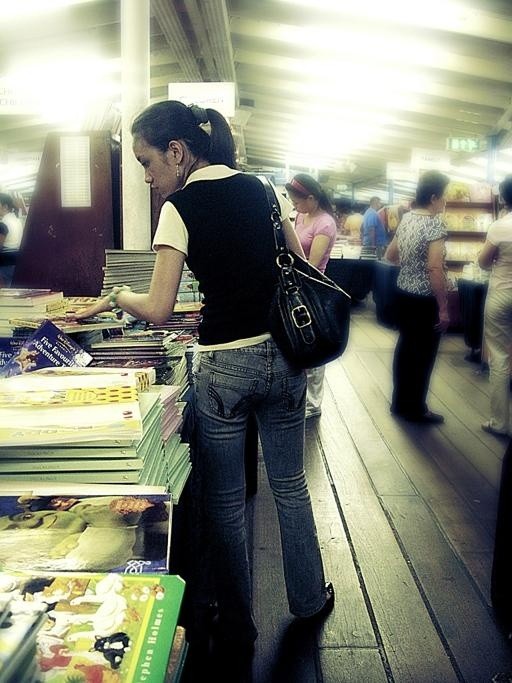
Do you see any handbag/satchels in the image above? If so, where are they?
[254,174,352,370]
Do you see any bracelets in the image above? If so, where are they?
[108,283,134,315]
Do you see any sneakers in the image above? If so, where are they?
[482,421,491,431]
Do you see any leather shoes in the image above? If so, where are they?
[421,410,444,424]
[321,582,334,611]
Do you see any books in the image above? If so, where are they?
[0,223,223,683]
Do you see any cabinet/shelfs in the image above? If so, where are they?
[446,192,498,289]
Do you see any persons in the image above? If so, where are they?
[60,100,333,644]
[488,440,512,682]
[0,191,17,220]
[288,169,511,434]
